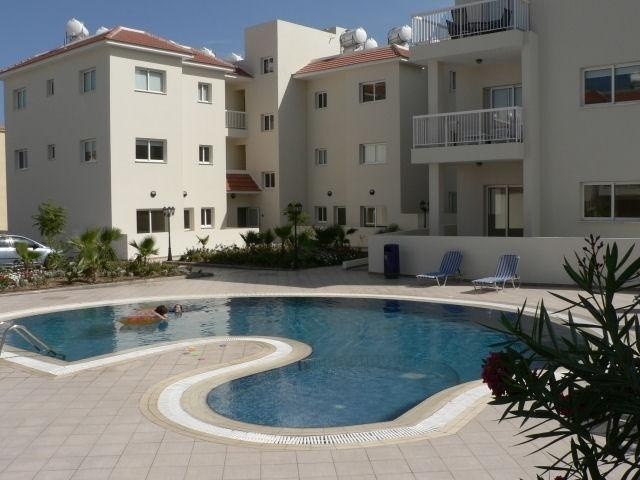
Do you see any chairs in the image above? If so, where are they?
[441,6,512,39]
[417,249,523,295]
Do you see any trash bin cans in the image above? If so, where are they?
[383,243,400,279]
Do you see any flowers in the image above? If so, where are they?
[482,249,640,480]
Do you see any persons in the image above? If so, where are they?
[173,305,183,315]
[135,306,170,321]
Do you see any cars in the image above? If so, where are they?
[0,234,60,271]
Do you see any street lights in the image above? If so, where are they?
[288,202,303,270]
[420,199,429,229]
[161,206,175,261]
